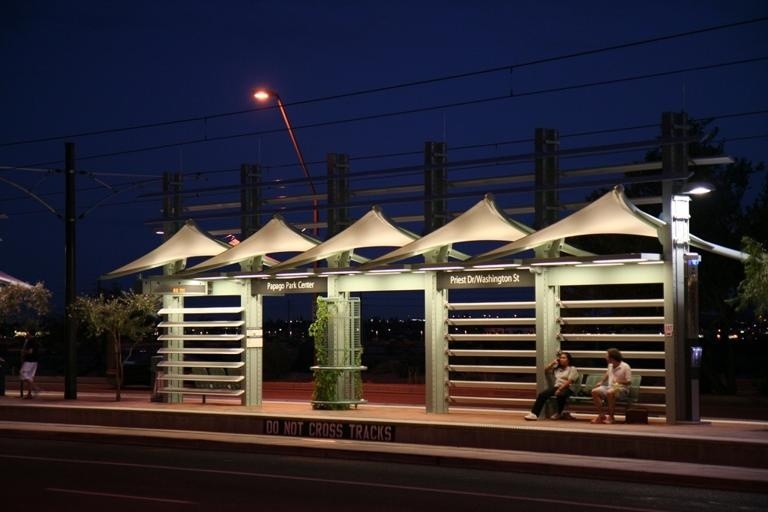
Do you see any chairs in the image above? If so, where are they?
[191,367,242,404]
[545,367,641,418]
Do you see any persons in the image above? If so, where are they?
[0,334,9,396]
[591,348,633,424]
[17,327,41,400]
[524,351,579,420]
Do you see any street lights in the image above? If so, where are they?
[253,85,322,377]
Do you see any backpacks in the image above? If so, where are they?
[569,370,582,395]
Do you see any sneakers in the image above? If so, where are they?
[524,412,537,421]
[591,415,615,424]
[550,412,561,420]
